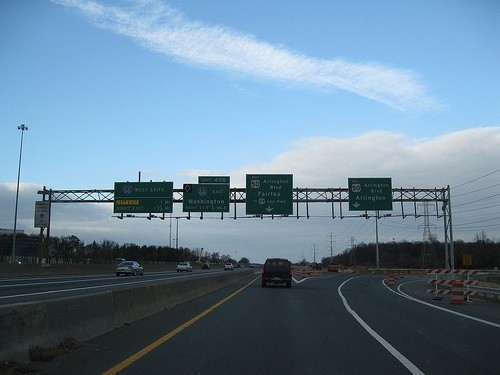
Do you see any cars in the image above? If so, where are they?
[115,261,144,277]
[202,263,210,270]
[224,263,234,271]
[176,262,192,272]
[235,263,260,269]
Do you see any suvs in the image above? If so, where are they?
[262,257,292,288]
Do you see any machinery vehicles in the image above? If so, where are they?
[327,259,339,272]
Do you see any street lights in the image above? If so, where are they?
[11,123,29,259]
